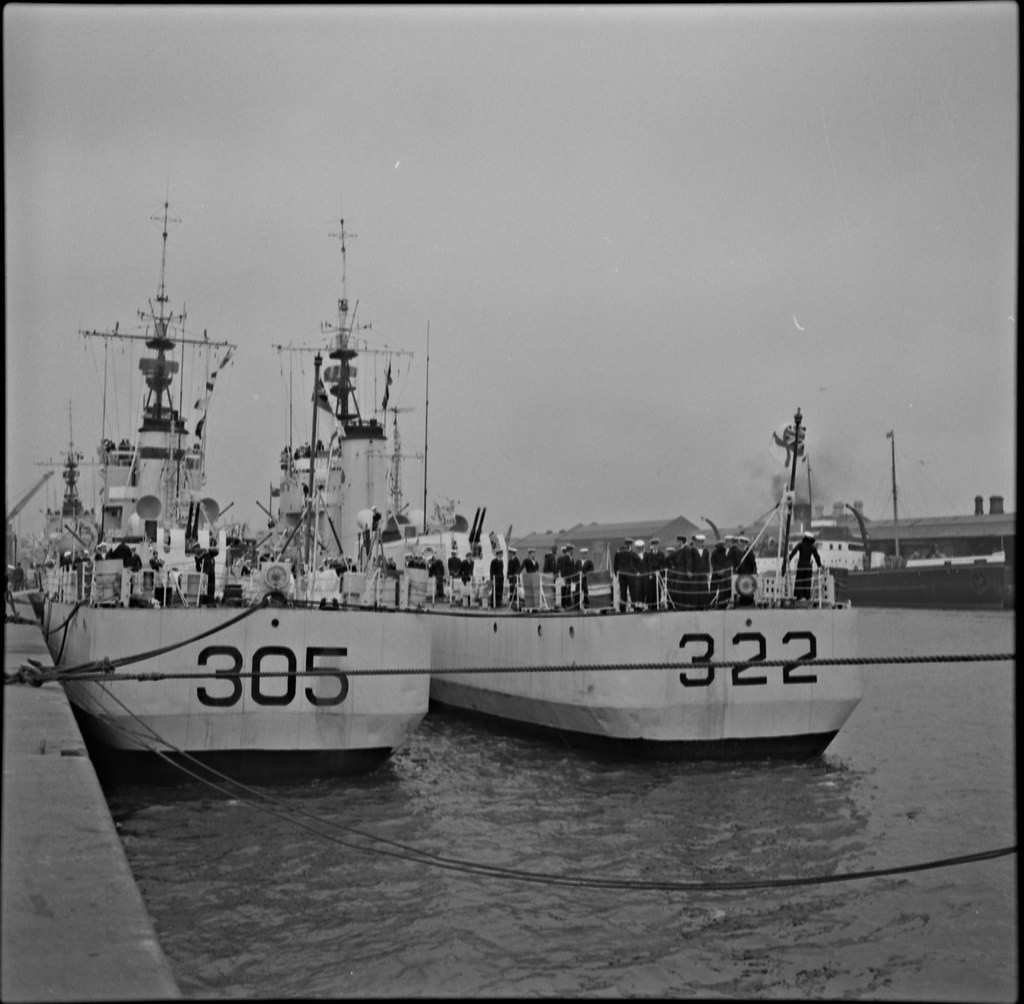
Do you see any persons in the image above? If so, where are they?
[488,548,506,609]
[12,561,25,591]
[278,543,476,609]
[573,546,595,610]
[543,546,559,613]
[611,529,759,613]
[148,550,166,576]
[505,545,521,610]
[785,531,824,605]
[33,536,143,609]
[555,547,574,612]
[517,547,540,613]
[190,542,218,608]
[559,543,575,612]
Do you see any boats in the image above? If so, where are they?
[413,412,864,760]
[38,196,440,759]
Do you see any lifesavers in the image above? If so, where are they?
[420,545,437,561]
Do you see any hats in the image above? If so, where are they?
[322,532,817,565]
[6,542,253,572]
